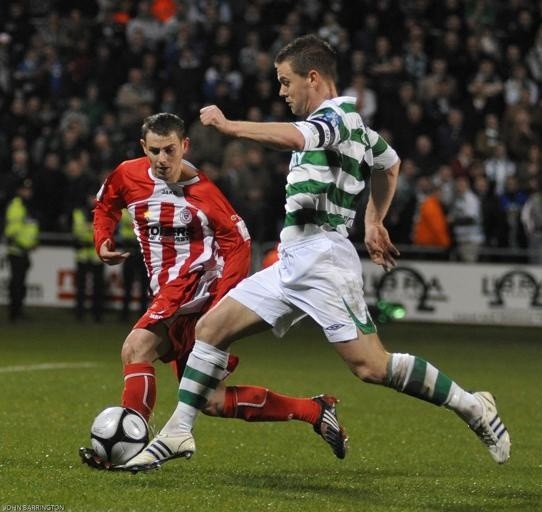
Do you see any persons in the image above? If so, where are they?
[122,32,512,474]
[1,0,542,266]
[4,173,40,325]
[114,202,150,325]
[71,179,106,327]
[77,111,350,473]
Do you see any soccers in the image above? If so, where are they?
[91,406,148,463]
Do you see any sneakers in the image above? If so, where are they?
[311,393,347,461]
[123,431,197,472]
[79,446,123,472]
[468,390,512,465]
